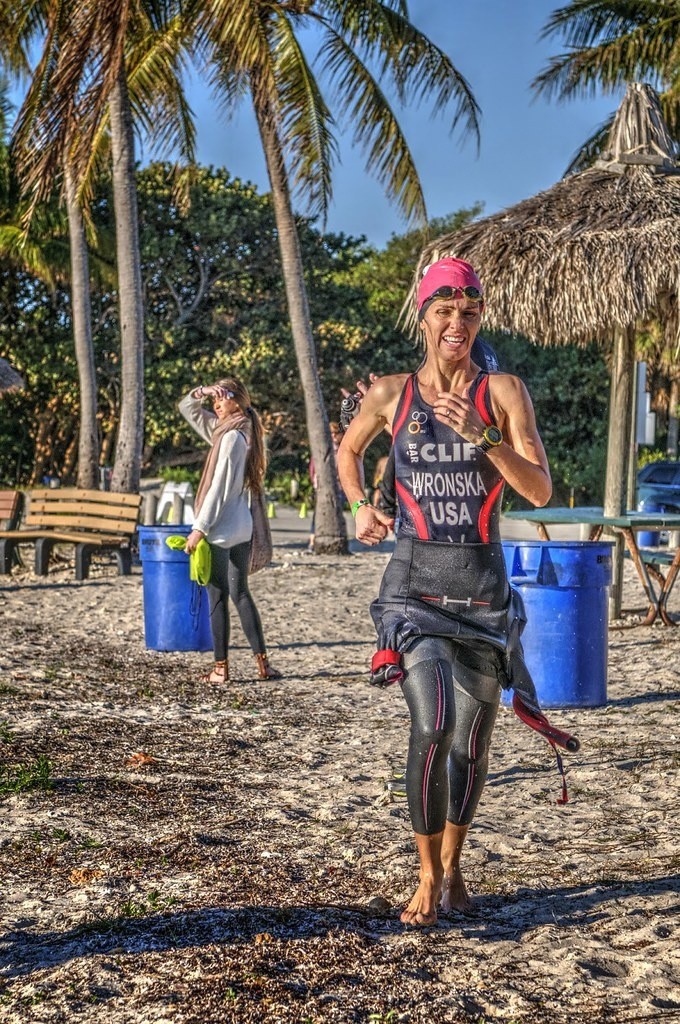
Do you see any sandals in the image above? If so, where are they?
[254,653,282,679]
[198,658,231,683]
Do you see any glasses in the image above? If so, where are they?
[227,392,238,400]
[422,285,484,309]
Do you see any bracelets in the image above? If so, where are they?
[352,499,369,518]
[195,385,206,396]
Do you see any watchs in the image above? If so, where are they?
[475,425,504,454]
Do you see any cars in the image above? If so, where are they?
[635,462,680,544]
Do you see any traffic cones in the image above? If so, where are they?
[267,503,276,518]
[299,503,308,518]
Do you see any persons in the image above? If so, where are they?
[305,420,389,551]
[335,258,551,924]
[179,377,283,683]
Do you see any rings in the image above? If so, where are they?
[446,408,452,416]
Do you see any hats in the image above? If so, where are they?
[418,258,484,314]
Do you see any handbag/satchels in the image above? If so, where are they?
[165,534,212,586]
